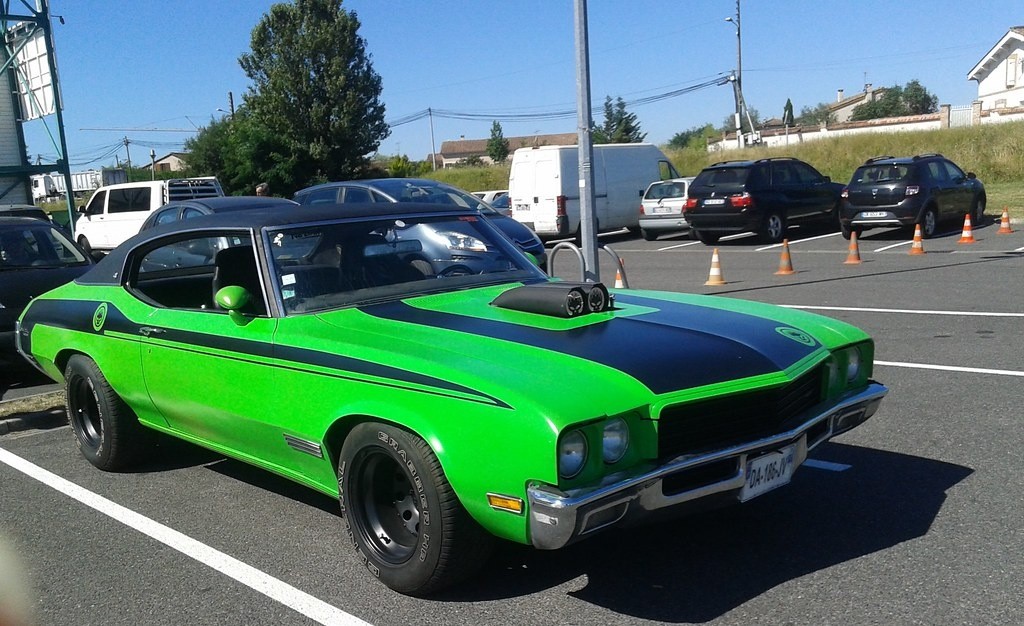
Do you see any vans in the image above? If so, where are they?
[637,176,698,241]
[74,175,227,259]
[506,142,685,250]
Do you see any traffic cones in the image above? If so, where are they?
[703,247,728,285]
[615,258,627,289]
[908,223,927,255]
[843,231,863,265]
[996,205,1014,234]
[957,214,976,243]
[772,238,800,275]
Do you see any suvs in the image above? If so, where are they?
[680,155,849,246]
[837,152,987,241]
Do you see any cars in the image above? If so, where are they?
[134,194,346,287]
[288,175,546,280]
[13,191,890,599]
[466,189,509,218]
[0,215,102,374]
[0,203,63,262]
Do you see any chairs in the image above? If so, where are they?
[888,166,900,178]
[340,236,423,290]
[213,243,279,316]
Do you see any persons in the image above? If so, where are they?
[350,231,394,289]
[255,182,271,198]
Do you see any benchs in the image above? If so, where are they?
[136,261,342,302]
